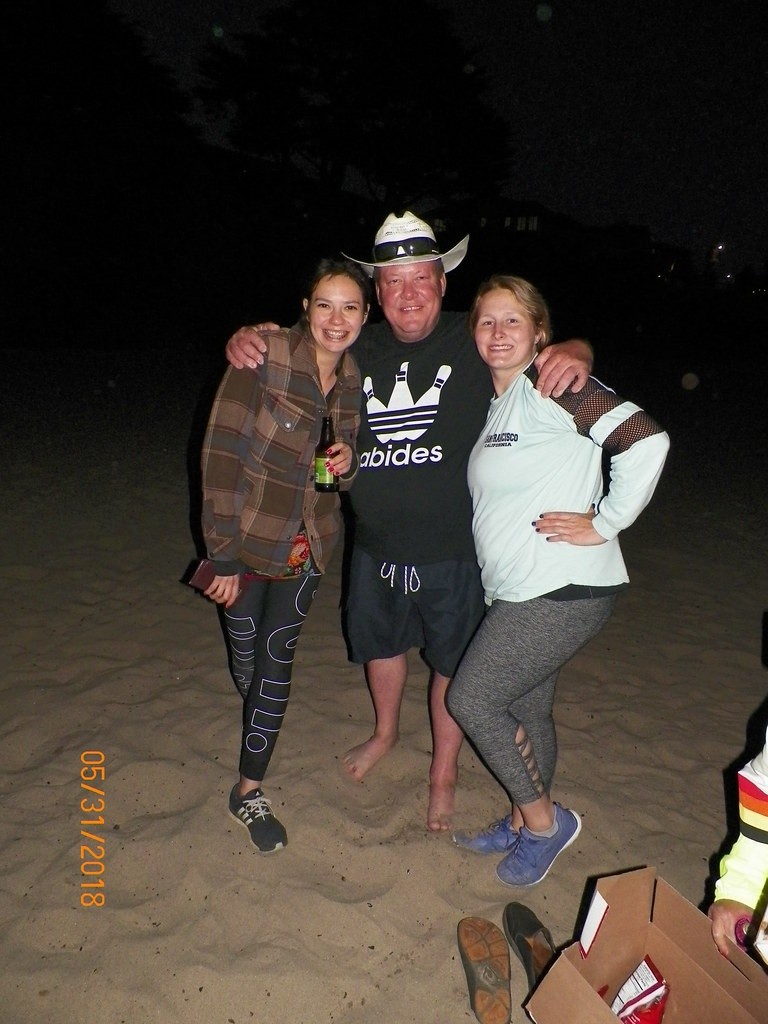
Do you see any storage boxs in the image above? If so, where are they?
[526,865,768,1024]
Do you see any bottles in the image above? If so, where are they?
[315,416,339,492]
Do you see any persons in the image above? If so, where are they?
[443,272,671,890]
[225,211,593,832]
[707,724,768,965]
[200,255,374,854]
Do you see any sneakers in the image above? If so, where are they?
[227,783,288,856]
[495,802,583,889]
[451,813,521,856]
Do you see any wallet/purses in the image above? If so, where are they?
[188,559,249,608]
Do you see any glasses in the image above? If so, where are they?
[373,237,440,262]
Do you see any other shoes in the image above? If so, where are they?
[457,916,512,1024]
[503,902,558,997]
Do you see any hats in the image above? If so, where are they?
[340,211,470,278]
